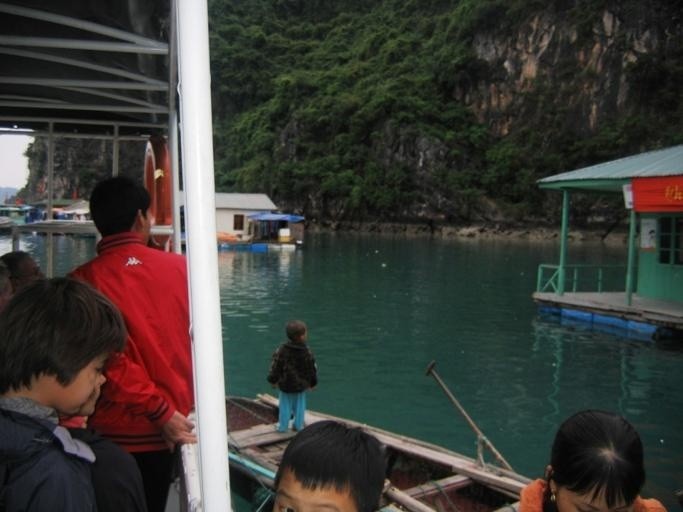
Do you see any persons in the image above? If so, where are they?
[517,408,667,512]
[266,320,319,432]
[0,251,198,454]
[65,174,195,512]
[271,418,385,512]
[1,274,129,512]
[58,370,148,512]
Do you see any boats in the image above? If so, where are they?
[529,145,682,343]
[225,392,544,512]
[20,193,306,253]
